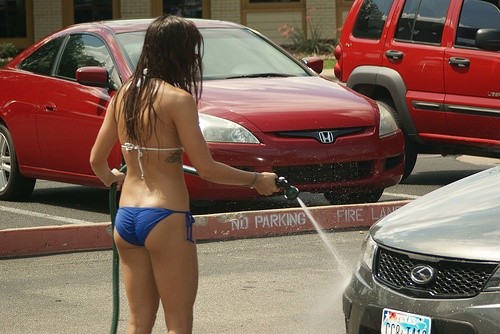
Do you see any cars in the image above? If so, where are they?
[342,163,500,334]
[0,18,410,205]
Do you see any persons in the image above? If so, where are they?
[89,15,283,334]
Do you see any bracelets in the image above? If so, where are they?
[247,172,259,188]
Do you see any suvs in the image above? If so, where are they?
[333,0,499,182]
